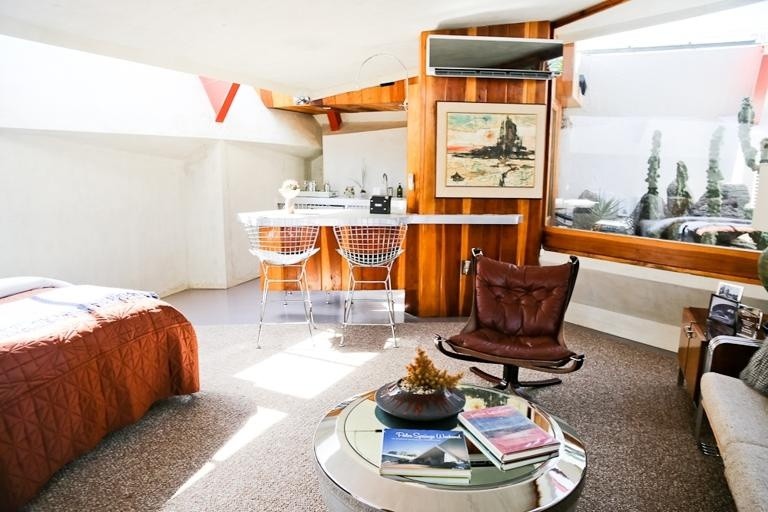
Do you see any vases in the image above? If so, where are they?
[375,380,466,419]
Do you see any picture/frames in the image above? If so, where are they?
[434,100,549,201]
[707,280,762,331]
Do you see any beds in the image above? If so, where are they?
[0,275,200,512]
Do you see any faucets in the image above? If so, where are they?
[382,171,388,196]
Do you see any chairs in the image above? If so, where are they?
[242,208,408,352]
[433,249,585,391]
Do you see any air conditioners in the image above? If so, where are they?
[425,34,564,78]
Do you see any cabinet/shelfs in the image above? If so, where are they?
[676,305,766,406]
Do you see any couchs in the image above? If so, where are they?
[693,332,768,512]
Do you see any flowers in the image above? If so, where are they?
[406,347,465,391]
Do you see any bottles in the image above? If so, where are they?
[397,185,403,198]
[324,181,330,192]
[388,186,393,196]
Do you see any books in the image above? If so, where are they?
[457,407,560,465]
[380,427,472,478]
[456,422,559,472]
[380,474,472,488]
